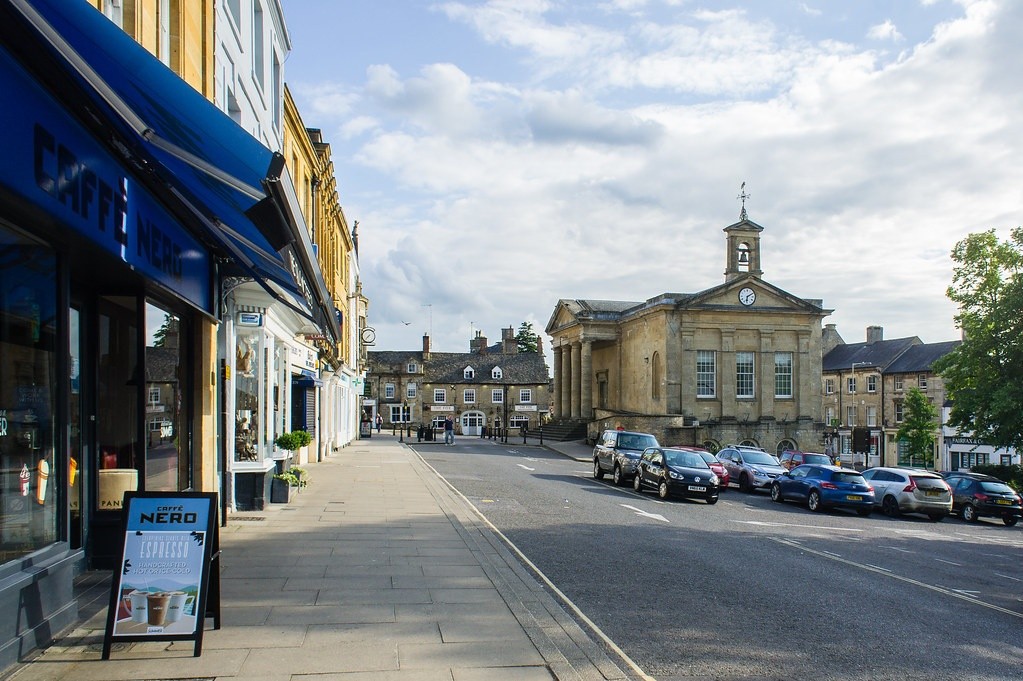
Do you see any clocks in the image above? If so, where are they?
[739,287,757,307]
[363,330,376,343]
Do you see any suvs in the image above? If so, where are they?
[593,430,662,486]
[778,448,832,473]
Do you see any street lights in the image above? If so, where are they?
[850,361,872,469]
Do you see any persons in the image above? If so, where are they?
[2,364,53,434]
[376,414,383,433]
[362,410,367,420]
[235,409,241,425]
[236,418,247,437]
[443,414,455,447]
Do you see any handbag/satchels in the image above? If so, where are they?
[379,417,382,424]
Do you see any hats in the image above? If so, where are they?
[362,410,365,414]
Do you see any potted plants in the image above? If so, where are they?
[272,433,302,503]
[289,430,312,487]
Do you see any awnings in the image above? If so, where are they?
[10,0,341,343]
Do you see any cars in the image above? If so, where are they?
[940,471,1023,528]
[770,463,876,516]
[715,446,790,493]
[673,446,729,492]
[860,467,953,522]
[634,447,720,504]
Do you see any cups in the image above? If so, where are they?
[146,593,171,625]
[36,470,48,504]
[19,478,29,496]
[69,457,77,487]
[166,591,195,621]
[123,591,149,623]
